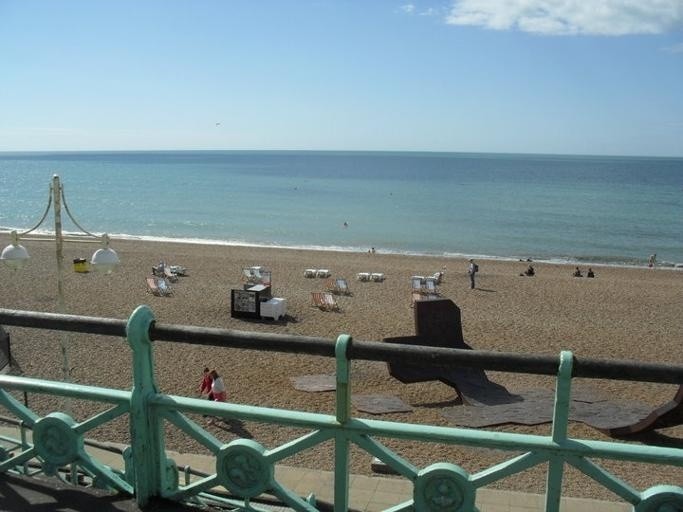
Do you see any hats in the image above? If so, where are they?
[468,259,474,262]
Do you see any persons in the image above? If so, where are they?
[574,266,594,277]
[524,264,535,276]
[648,254,657,272]
[200,367,226,426]
[467,257,478,289]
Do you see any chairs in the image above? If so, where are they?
[304,269,317,278]
[322,292,343,310]
[425,278,439,292]
[336,279,350,295]
[357,273,370,281]
[409,292,427,308]
[316,270,331,278]
[427,292,445,301]
[372,273,385,282]
[310,291,324,307]
[242,266,262,280]
[145,258,187,298]
[411,278,425,292]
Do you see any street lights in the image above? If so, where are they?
[0,171,125,490]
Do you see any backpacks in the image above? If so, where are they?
[475,265,478,272]
